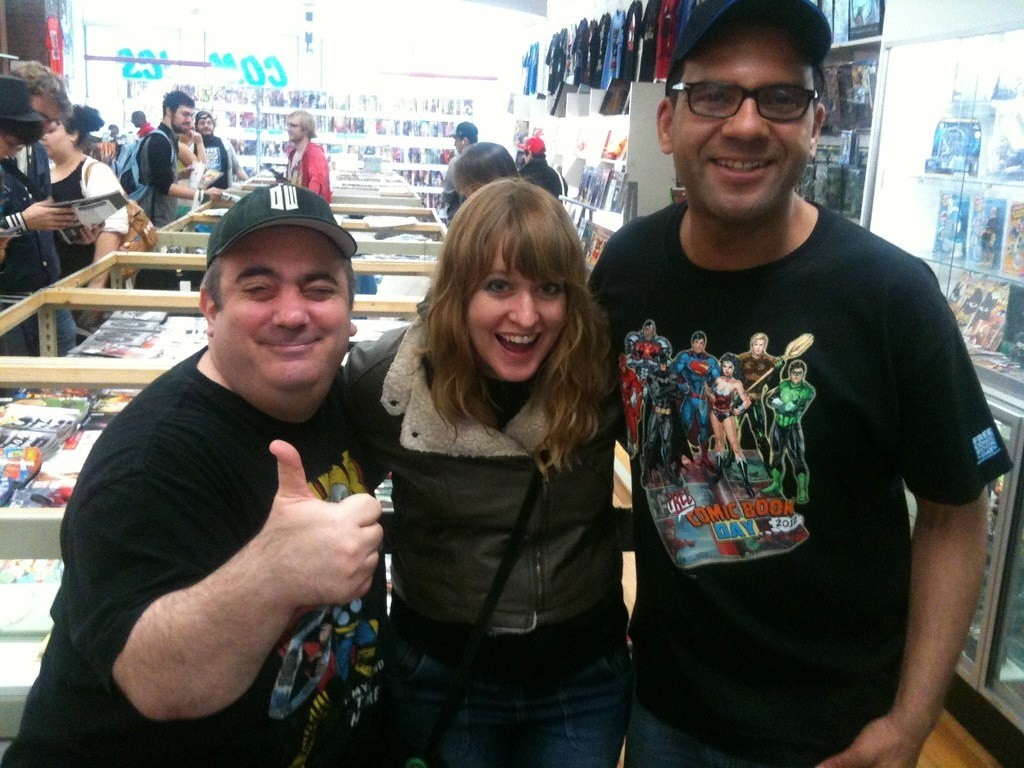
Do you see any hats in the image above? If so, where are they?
[517,137,545,154]
[672,1,831,67]
[206,184,357,268]
[0,74,44,122]
[451,122,478,138]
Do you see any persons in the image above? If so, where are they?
[442,122,479,222]
[341,177,629,768]
[0,183,389,768]
[285,110,331,205]
[452,142,520,198]
[584,0,1014,768]
[0,62,248,357]
[517,137,567,207]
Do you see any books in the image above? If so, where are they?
[0,310,211,696]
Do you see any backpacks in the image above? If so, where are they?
[111,130,174,202]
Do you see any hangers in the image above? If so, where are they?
[529,0,640,47]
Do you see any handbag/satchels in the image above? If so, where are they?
[114,201,158,277]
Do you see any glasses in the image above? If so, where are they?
[286,123,303,128]
[668,81,819,122]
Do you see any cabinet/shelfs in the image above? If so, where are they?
[908,388,1024,733]
[869,20,1021,406]
